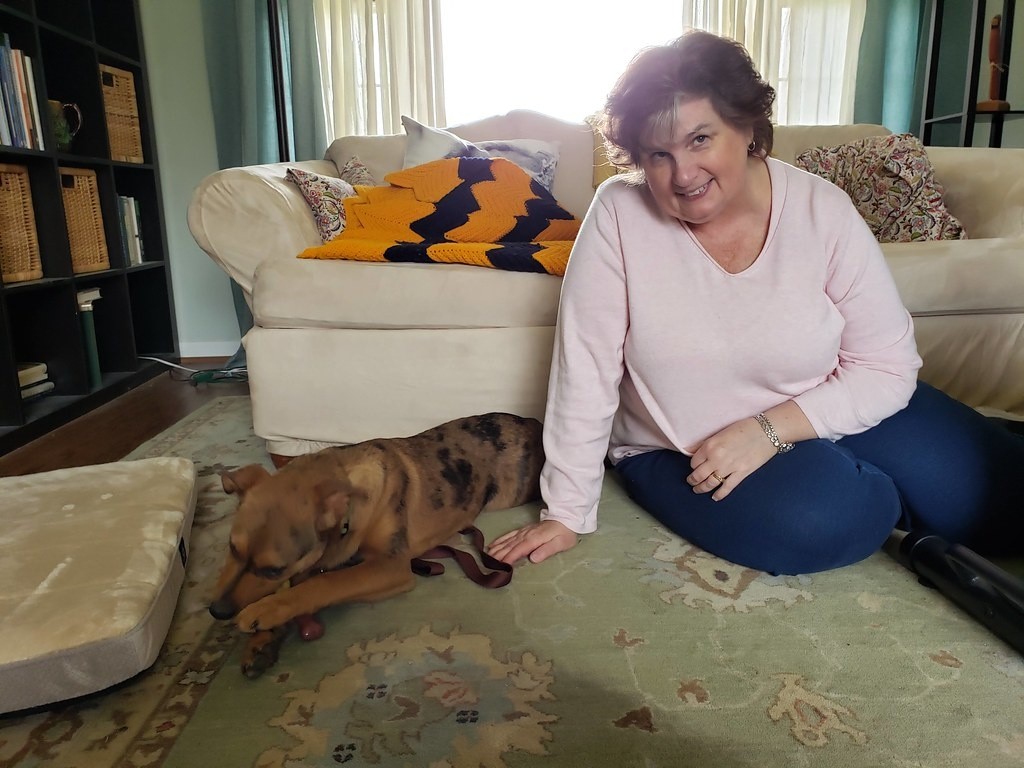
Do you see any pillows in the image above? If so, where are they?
[795,134,970,244]
[283,154,380,242]
[401,115,561,192]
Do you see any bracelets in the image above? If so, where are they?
[755,413,794,454]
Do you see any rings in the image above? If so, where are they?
[713,474,723,482]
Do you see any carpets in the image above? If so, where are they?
[0,398,1024,768]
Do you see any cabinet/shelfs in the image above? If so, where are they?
[0,0,181,458]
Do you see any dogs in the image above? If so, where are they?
[205,409,546,635]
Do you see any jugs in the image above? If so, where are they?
[48,100,82,144]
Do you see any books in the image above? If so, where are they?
[18,363,54,398]
[116,195,146,267]
[0,33,45,152]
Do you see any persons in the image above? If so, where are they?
[487,29,1024,575]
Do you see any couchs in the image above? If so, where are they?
[186,123,1024,473]
